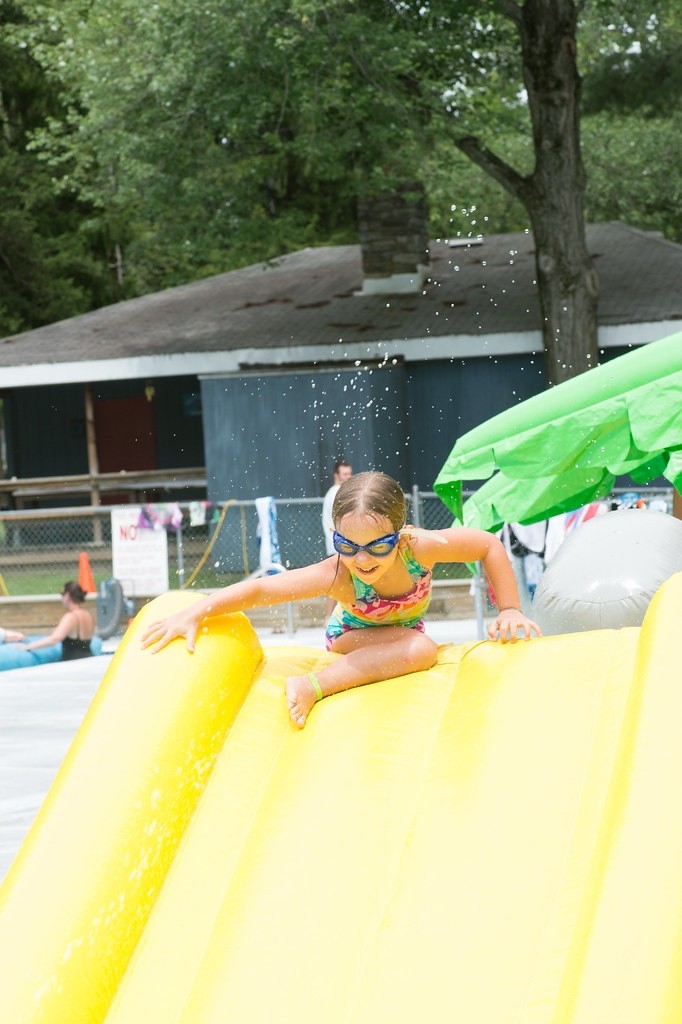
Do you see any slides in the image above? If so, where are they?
[1,571,681,1024]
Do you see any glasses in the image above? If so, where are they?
[334,531,399,557]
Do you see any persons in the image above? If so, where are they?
[19,579,94,665]
[321,461,353,560]
[141,471,543,731]
